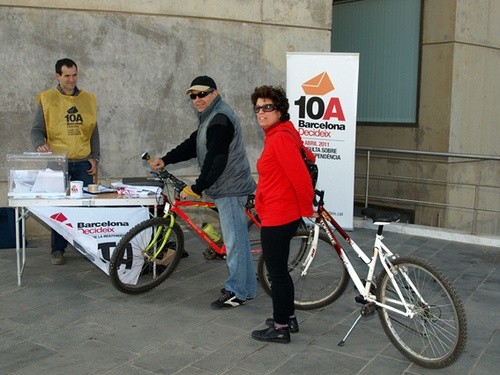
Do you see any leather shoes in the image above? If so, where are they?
[265,317,299,333]
[251,324,290,343]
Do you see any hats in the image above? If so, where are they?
[185,76,216,95]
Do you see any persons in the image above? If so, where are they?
[152,75,257,310]
[251,85,320,344]
[31,59,100,264]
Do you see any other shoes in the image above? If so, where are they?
[51,251,64,265]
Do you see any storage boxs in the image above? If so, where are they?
[6,152,69,198]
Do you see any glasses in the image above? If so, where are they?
[254,104,278,113]
[190,90,214,100]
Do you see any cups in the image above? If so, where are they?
[69,181,83,197]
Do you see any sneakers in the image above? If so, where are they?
[210,291,246,308]
[222,287,252,300]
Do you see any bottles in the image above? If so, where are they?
[201,222,221,242]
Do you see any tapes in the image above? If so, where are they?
[88,185,99,192]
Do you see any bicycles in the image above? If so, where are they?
[109,166,309,295]
[257,188,469,369]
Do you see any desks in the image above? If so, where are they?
[8,189,164,287]
